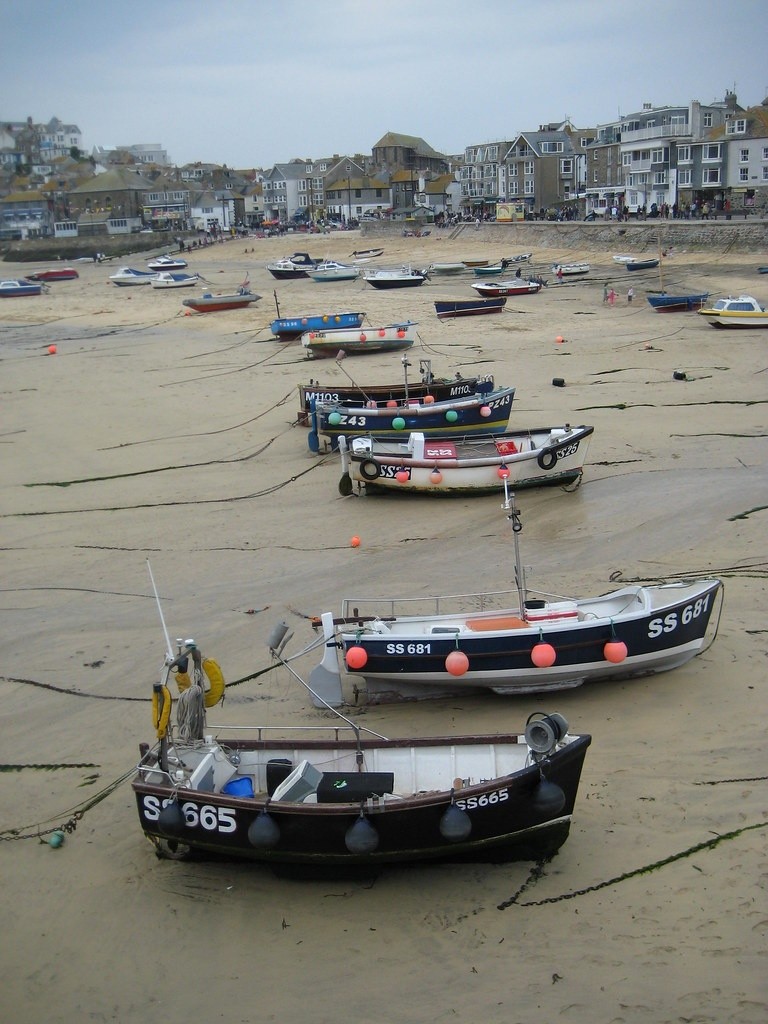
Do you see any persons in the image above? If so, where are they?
[557,269,563,284]
[435,211,488,228]
[501,258,509,275]
[93,252,105,262]
[515,268,522,278]
[302,216,338,229]
[603,284,633,306]
[524,192,730,221]
[179,220,279,254]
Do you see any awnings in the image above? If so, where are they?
[603,193,614,198]
[614,192,624,197]
[459,197,535,206]
[585,193,599,198]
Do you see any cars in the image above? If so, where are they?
[327,220,342,228]
[230,223,247,232]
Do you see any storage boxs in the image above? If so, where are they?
[224,777,256,800]
[524,601,579,627]
[270,759,324,802]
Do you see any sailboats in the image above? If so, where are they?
[646,236,709,313]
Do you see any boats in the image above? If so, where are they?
[131,638,592,867]
[757,267,768,274]
[267,252,363,282]
[431,263,465,274]
[269,288,418,356]
[308,494,724,710]
[612,256,660,270]
[434,276,549,318]
[296,357,494,427]
[0,255,263,312]
[697,295,768,329]
[308,381,515,454]
[461,253,533,274]
[362,264,431,289]
[552,263,590,275]
[337,422,594,496]
[348,247,385,259]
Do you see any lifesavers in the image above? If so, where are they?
[537,447,558,470]
[360,459,382,481]
